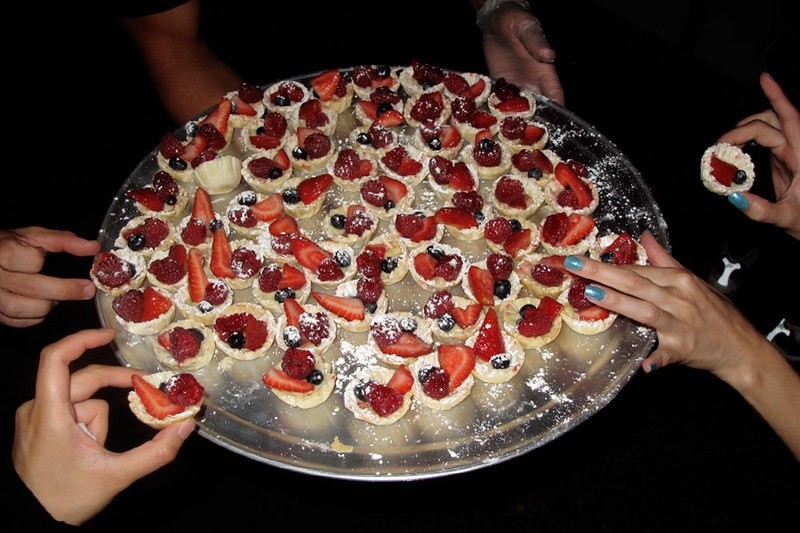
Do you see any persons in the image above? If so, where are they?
[569,66,800,466]
[0,221,200,532]
[120,0,571,128]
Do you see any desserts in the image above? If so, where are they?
[90,59,651,431]
[699,142,756,197]
